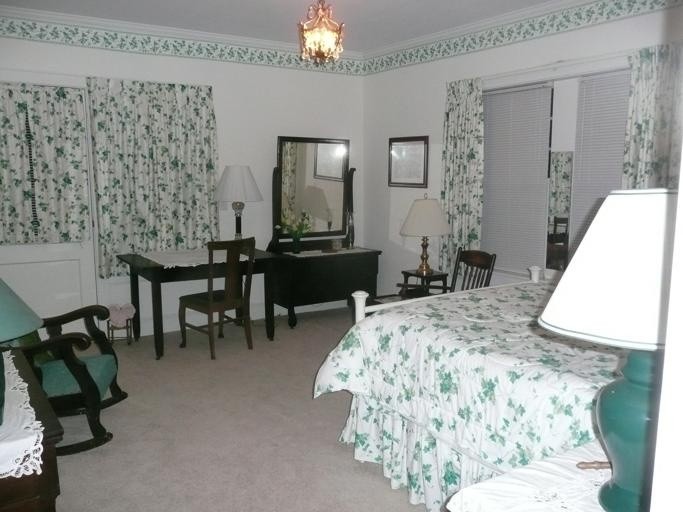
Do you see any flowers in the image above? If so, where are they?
[275,193,312,238]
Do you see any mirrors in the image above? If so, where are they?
[270,136,356,253]
[546,151,573,270]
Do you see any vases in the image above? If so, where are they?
[293,238,301,253]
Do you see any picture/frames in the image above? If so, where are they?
[388,136,428,189]
[313,143,343,181]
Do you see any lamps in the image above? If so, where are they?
[538,187,679,512]
[297,0,344,66]
[213,166,263,241]
[0,279,43,343]
[400,193,453,276]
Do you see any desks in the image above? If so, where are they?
[117,248,276,361]
[403,270,449,295]
[265,248,382,341]
[0,350,65,512]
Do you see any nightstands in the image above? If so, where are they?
[448,438,613,512]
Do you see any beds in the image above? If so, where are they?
[312,266,629,512]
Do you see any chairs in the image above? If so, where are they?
[180,236,255,359]
[0,306,128,456]
[450,247,496,292]
[546,218,567,263]
[107,319,132,344]
